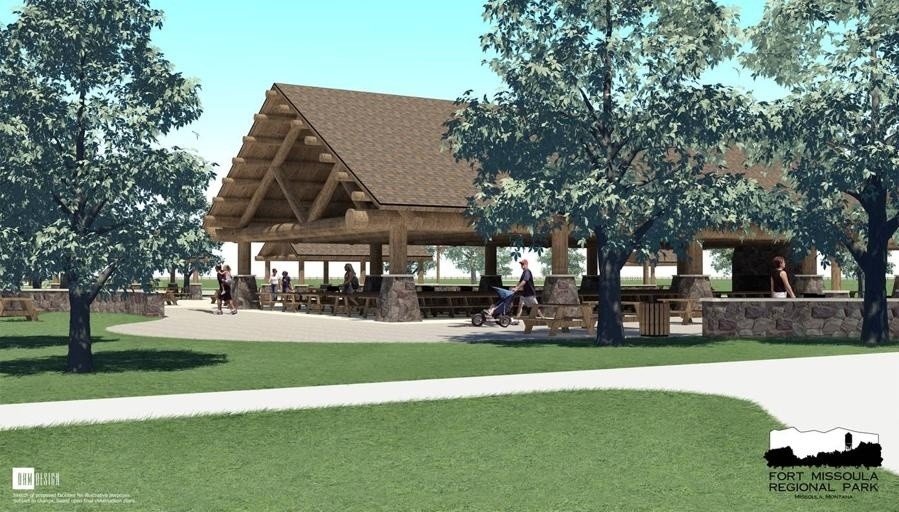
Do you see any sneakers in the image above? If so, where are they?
[214,309,236,314]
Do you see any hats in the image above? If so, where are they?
[519,260,528,266]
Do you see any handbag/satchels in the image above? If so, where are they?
[351,276,359,289]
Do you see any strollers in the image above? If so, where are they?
[469,285,518,328]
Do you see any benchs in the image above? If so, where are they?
[31,304,45,321]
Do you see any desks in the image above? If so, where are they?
[0,296,38,321]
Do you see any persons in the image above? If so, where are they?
[770,256,796,299]
[511,259,544,319]
[486,304,497,313]
[219,265,232,295]
[338,263,360,306]
[282,271,291,293]
[268,269,280,300]
[215,264,237,314]
